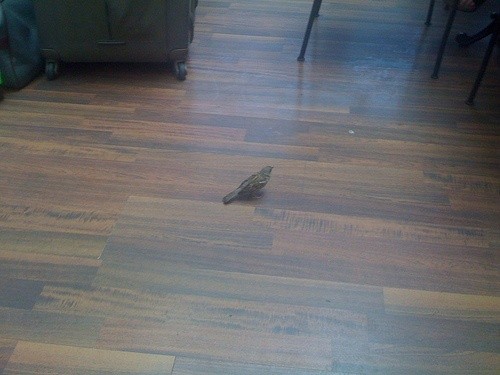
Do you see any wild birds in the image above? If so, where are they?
[223,165,274,205]
